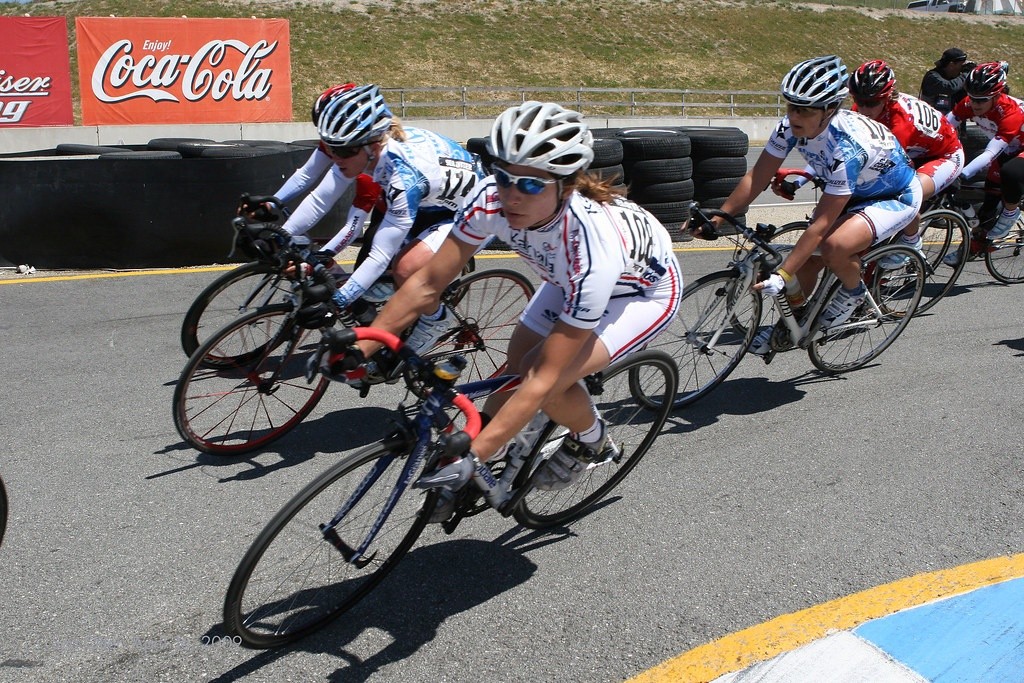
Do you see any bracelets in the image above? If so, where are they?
[777,268,793,283]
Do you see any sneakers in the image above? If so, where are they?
[531,418,607,492]
[426,488,482,524]
[366,362,399,384]
[821,279,868,328]
[987,208,1022,239]
[877,235,923,269]
[405,304,458,358]
[748,325,773,355]
[943,248,974,267]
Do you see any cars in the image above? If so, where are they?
[905,0,969,13]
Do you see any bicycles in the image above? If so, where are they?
[169,192,540,457]
[725,165,1023,350]
[627,201,929,412]
[216,309,680,650]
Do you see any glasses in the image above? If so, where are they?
[854,101,881,108]
[967,95,996,103]
[491,162,558,195]
[322,140,381,159]
[786,102,823,118]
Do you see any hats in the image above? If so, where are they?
[934,48,966,68]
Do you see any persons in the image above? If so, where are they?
[769,60,966,270]
[233,74,498,363]
[680,53,922,355]
[917,47,1024,266]
[322,98,684,525]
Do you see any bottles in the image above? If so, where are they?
[349,301,377,325]
[466,413,508,461]
[785,274,806,308]
[961,203,980,227]
[321,258,346,274]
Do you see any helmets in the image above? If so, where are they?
[848,60,897,101]
[965,62,1007,98]
[780,55,849,108]
[318,84,392,147]
[490,101,595,175]
[311,83,355,127]
